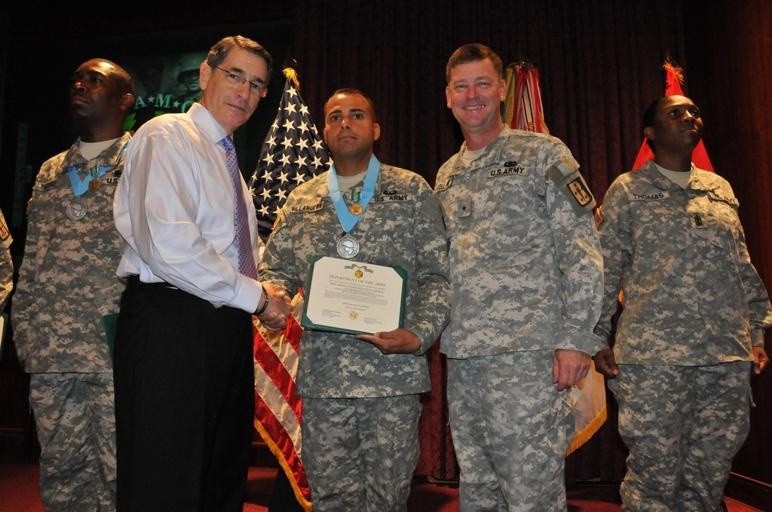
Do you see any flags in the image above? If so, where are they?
[498,56,608,457]
[246,74,341,512]
[629,63,716,172]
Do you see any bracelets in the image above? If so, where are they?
[255,285,270,316]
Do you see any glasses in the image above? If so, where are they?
[213,64,264,95]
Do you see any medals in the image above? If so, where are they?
[336,233,360,260]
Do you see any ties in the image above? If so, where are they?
[221,134,258,282]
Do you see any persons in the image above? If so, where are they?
[0,198,17,352]
[429,44,605,512]
[253,85,452,512]
[11,58,143,510]
[111,35,295,512]
[576,50,770,512]
